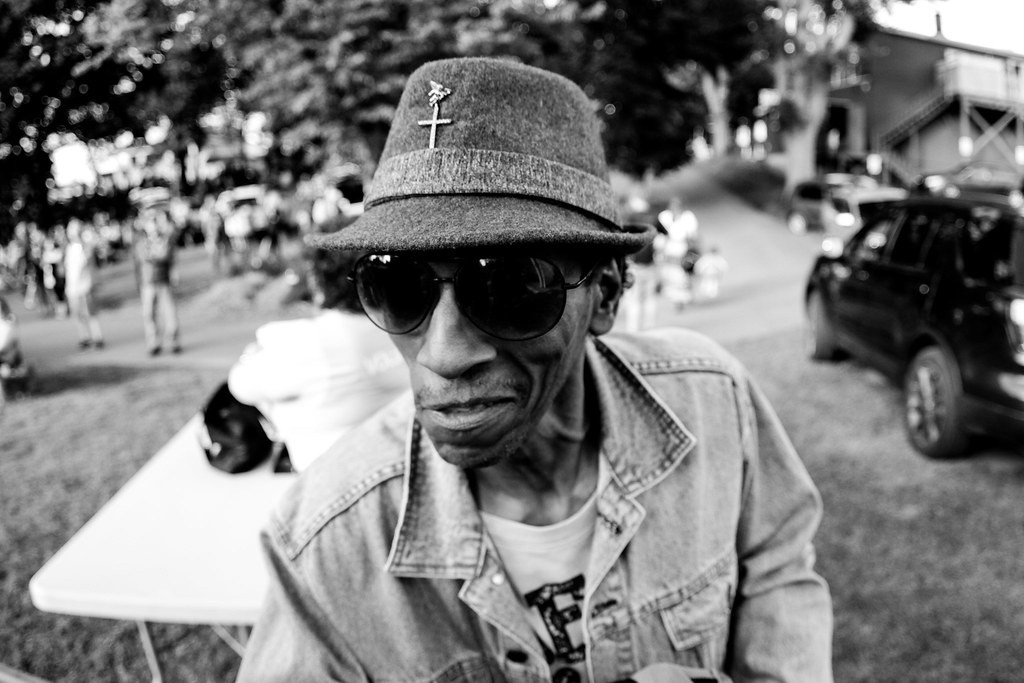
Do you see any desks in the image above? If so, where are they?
[29,407,302,683]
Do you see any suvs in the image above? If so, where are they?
[798,194,1020,457]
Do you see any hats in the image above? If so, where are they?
[302,54,657,259]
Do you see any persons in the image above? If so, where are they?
[0,160,366,398]
[234,57,833,683]
[628,198,720,330]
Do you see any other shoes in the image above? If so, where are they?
[151,344,160,357]
[80,341,107,348]
[171,345,180,354]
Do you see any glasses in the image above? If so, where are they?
[346,250,598,343]
